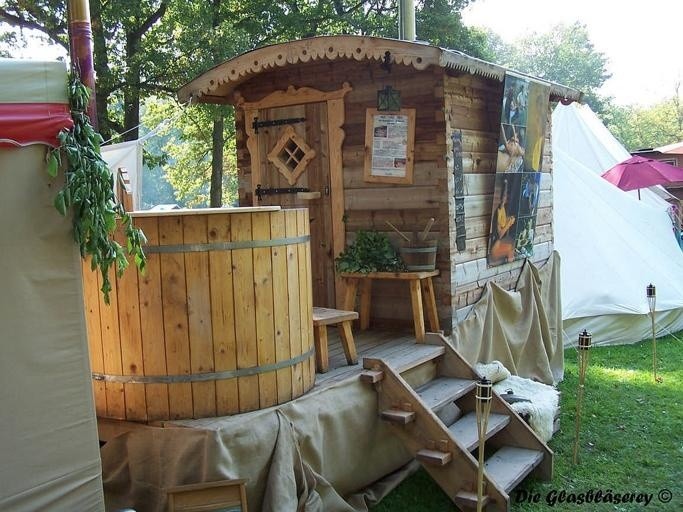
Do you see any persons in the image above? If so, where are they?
[489,77,538,266]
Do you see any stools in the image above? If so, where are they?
[312,307,360,375]
[340,269,441,344]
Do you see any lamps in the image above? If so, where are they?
[376,64,402,114]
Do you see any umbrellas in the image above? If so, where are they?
[599,155,683,202]
